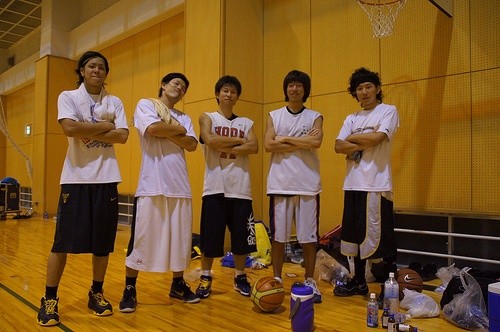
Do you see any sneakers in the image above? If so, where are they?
[377,285,384,308]
[169,281,200,303]
[38,295,60,326]
[333,278,368,295]
[196,276,211,297]
[118,285,137,312]
[88,289,113,316]
[304,277,322,302]
[233,274,251,295]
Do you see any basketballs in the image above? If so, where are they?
[395,268,423,302]
[249,277,285,312]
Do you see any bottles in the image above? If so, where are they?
[289,281,315,332]
[381,308,417,332]
[44,212,48,219]
[367,292,378,328]
[383,272,399,313]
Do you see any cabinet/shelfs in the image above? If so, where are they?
[393,206,500,279]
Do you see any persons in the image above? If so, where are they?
[264,70,323,303]
[334,66,400,304]
[119,73,200,312]
[37,50,131,326]
[195,75,259,298]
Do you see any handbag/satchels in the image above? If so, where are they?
[398,266,497,331]
